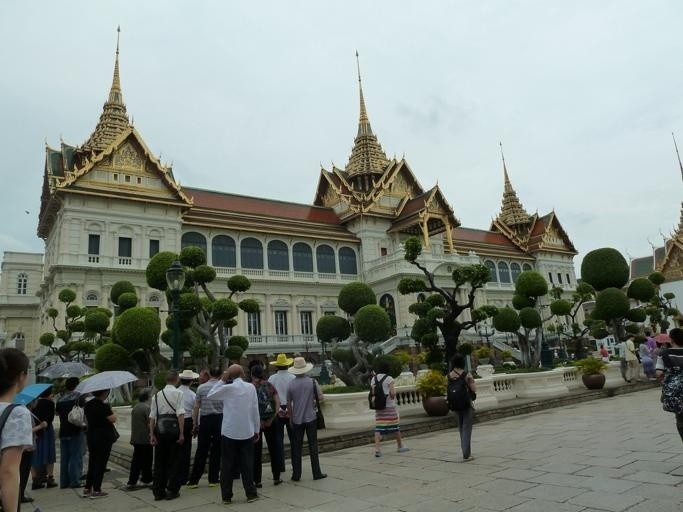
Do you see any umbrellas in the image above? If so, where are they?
[37,362,96,380]
[73,370,139,409]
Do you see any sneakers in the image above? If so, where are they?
[82,489,92,498]
[125,484,140,491]
[396,447,411,453]
[90,491,109,500]
[462,455,474,462]
[140,482,154,488]
[206,480,222,487]
[186,480,200,489]
[375,449,384,458]
[246,495,260,503]
[222,497,233,505]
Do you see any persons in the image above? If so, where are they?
[13,377,117,503]
[446,355,475,459]
[600,329,683,441]
[0,348,33,512]
[371,362,410,458]
[124,353,327,503]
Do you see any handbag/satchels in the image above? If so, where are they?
[100,424,120,446]
[156,412,181,437]
[469,390,477,401]
[315,411,326,430]
[67,405,88,428]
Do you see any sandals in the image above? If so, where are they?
[273,479,283,486]
[255,482,263,488]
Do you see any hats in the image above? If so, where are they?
[269,353,294,367]
[177,369,199,380]
[287,355,314,376]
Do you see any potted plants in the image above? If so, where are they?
[499,350,512,361]
[395,352,413,371]
[416,351,429,369]
[569,355,609,390]
[413,369,449,416]
[472,347,490,365]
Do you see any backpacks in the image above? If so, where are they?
[446,377,470,414]
[660,366,683,413]
[368,381,386,411]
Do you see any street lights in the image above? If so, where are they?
[164,254,187,376]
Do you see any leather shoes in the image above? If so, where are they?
[313,473,328,480]
[291,476,300,481]
[153,493,167,500]
[166,492,181,500]
